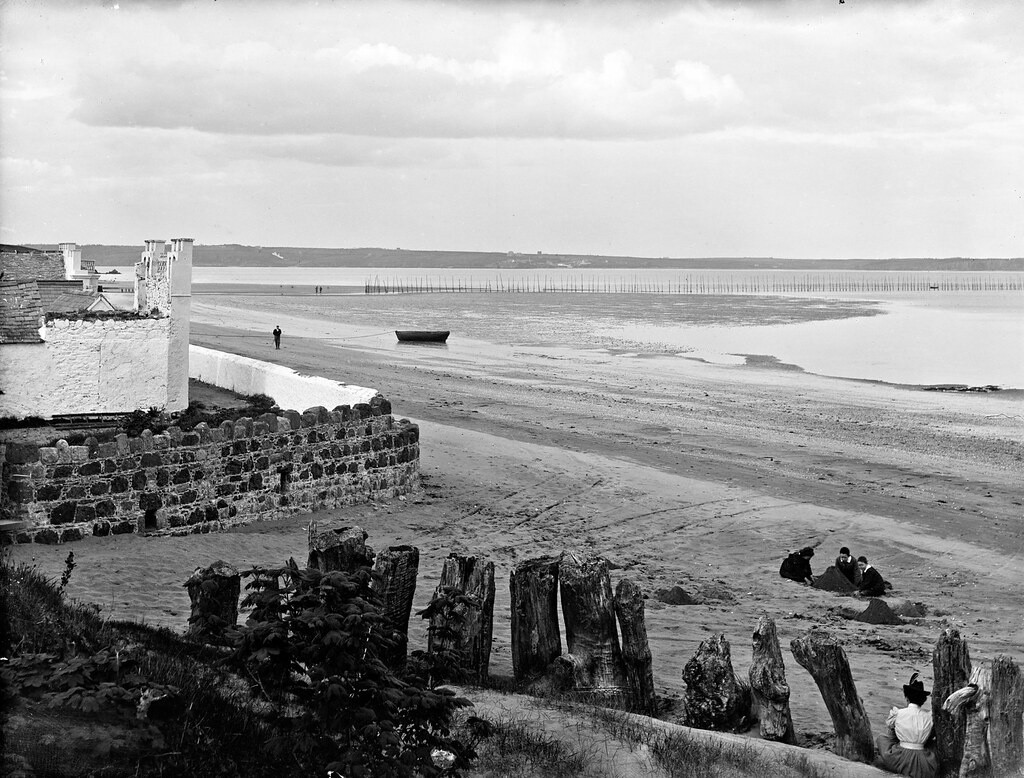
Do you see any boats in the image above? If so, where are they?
[395,330,450,341]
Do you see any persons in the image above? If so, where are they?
[779,547,815,583]
[856,556,885,596]
[273,326,282,349]
[877,681,937,778]
[835,547,861,586]
